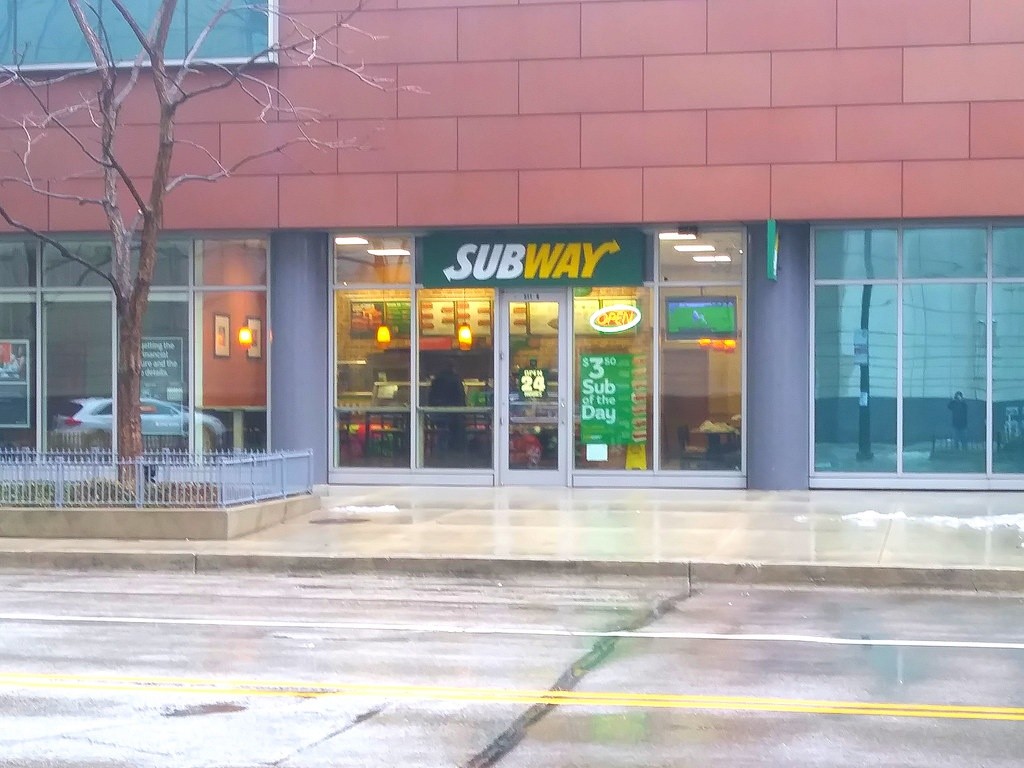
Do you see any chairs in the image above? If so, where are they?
[677,424,741,471]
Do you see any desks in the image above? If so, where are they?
[343,392,557,464]
[689,428,735,470]
[362,406,491,456]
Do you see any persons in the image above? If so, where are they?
[948,390,968,449]
[1,344,25,383]
[429,349,470,462]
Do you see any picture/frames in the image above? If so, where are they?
[213,312,231,358]
[248,318,262,359]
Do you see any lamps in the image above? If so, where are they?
[377,325,390,343]
[458,323,473,346]
[238,320,253,345]
